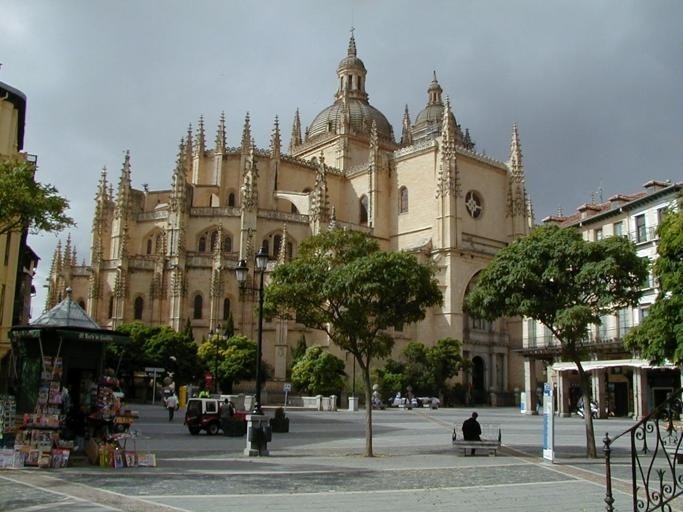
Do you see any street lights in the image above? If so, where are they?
[253,247,268,416]
[235,260,250,334]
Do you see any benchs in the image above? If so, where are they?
[452,428,502,457]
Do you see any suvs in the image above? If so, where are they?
[185,397,246,434]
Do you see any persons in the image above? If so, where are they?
[218,398,234,437]
[372,391,439,412]
[165,392,177,422]
[461,412,482,455]
[198,387,210,398]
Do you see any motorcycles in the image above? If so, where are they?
[576,397,597,419]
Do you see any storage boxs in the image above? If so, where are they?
[84,436,121,463]
